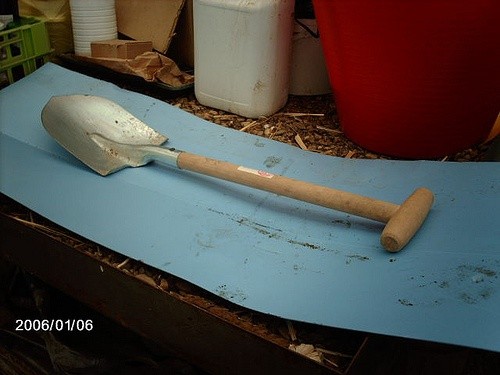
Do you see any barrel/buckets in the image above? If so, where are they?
[286,17,331,96]
[312,0,500,159]
[192,0,293,119]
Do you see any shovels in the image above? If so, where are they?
[38,93,435,256]
